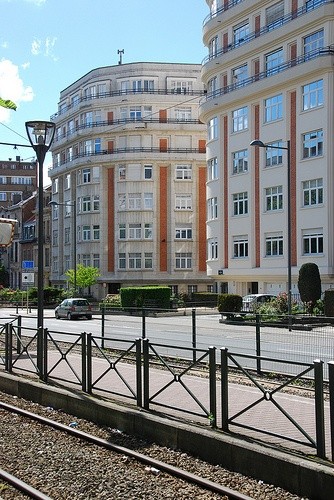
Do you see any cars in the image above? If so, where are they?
[240,294,278,316]
[53,298,93,321]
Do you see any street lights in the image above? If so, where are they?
[25,120,55,376]
[47,199,78,299]
[250,139,295,332]
